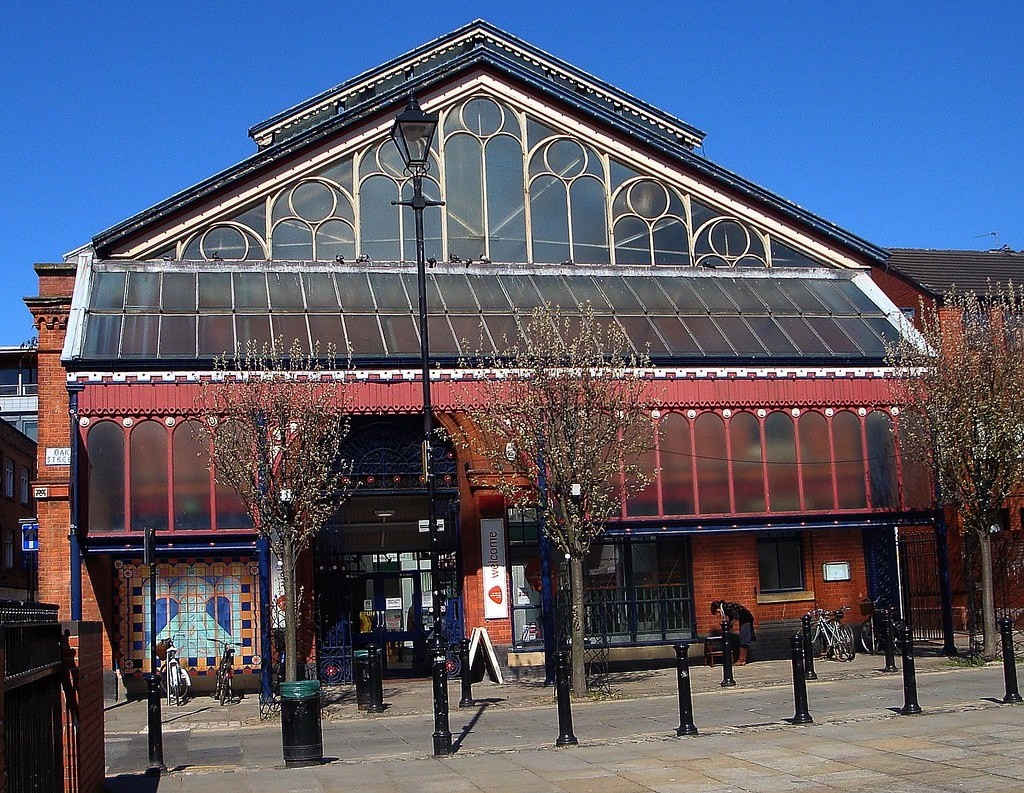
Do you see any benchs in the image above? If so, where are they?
[758,618,824,658]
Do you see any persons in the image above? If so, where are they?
[711,600,756,666]
[407,594,414,631]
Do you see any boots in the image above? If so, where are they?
[732,647,748,666]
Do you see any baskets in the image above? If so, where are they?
[859,602,874,616]
[157,642,168,661]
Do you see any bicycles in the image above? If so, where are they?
[206,638,242,706]
[795,606,855,662]
[156,633,192,706]
[859,594,914,656]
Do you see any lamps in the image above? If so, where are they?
[374,509,396,517]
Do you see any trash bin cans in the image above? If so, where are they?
[353,648,384,710]
[278,679,324,768]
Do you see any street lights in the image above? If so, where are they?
[389,90,453,753]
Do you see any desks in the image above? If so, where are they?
[704,636,734,668]
